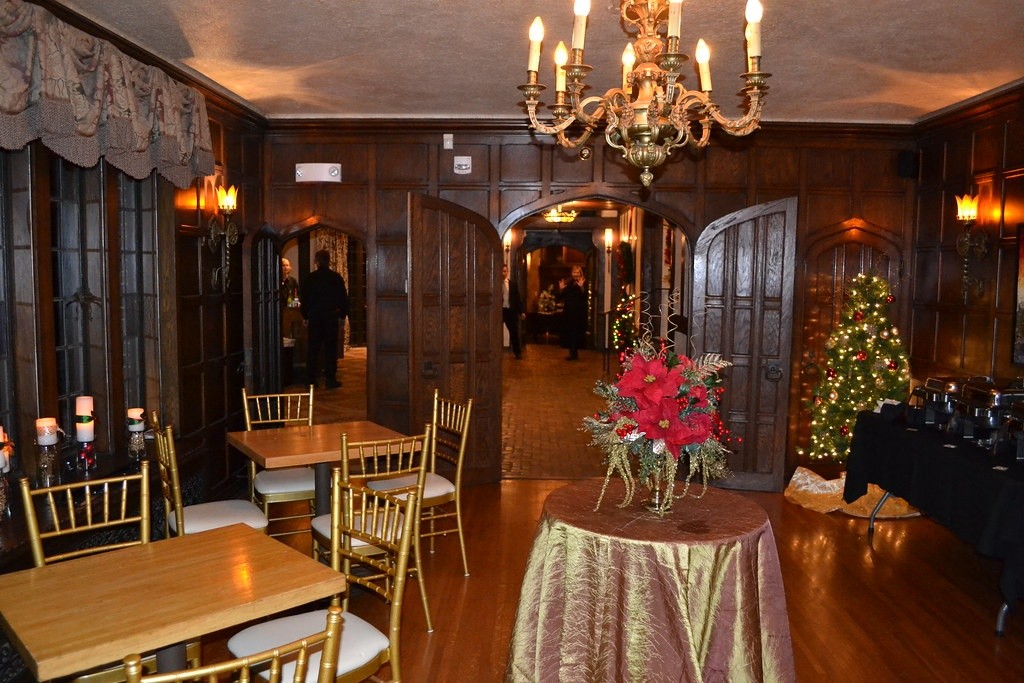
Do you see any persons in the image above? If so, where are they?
[282,258,298,299]
[301,250,348,388]
[503,265,526,360]
[556,266,589,361]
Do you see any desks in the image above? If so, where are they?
[843,403,1024,637]
[226,419,421,597]
[0,523,346,676]
[505,478,794,683]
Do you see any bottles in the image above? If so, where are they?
[287,288,301,307]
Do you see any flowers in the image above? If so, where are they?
[576,337,734,519]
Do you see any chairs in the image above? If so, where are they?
[18,384,473,683]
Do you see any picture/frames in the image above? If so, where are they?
[1010,223,1024,366]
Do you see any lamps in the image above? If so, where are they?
[504,229,511,266]
[954,194,989,262]
[605,228,613,273]
[542,205,580,225]
[517,0,773,188]
[209,184,239,253]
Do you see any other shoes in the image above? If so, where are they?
[567,355,578,360]
[305,381,319,389]
[327,381,342,388]
[515,351,521,358]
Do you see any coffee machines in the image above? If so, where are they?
[904,374,1024,463]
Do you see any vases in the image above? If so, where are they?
[642,459,670,511]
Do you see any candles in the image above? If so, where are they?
[0,396,145,473]
